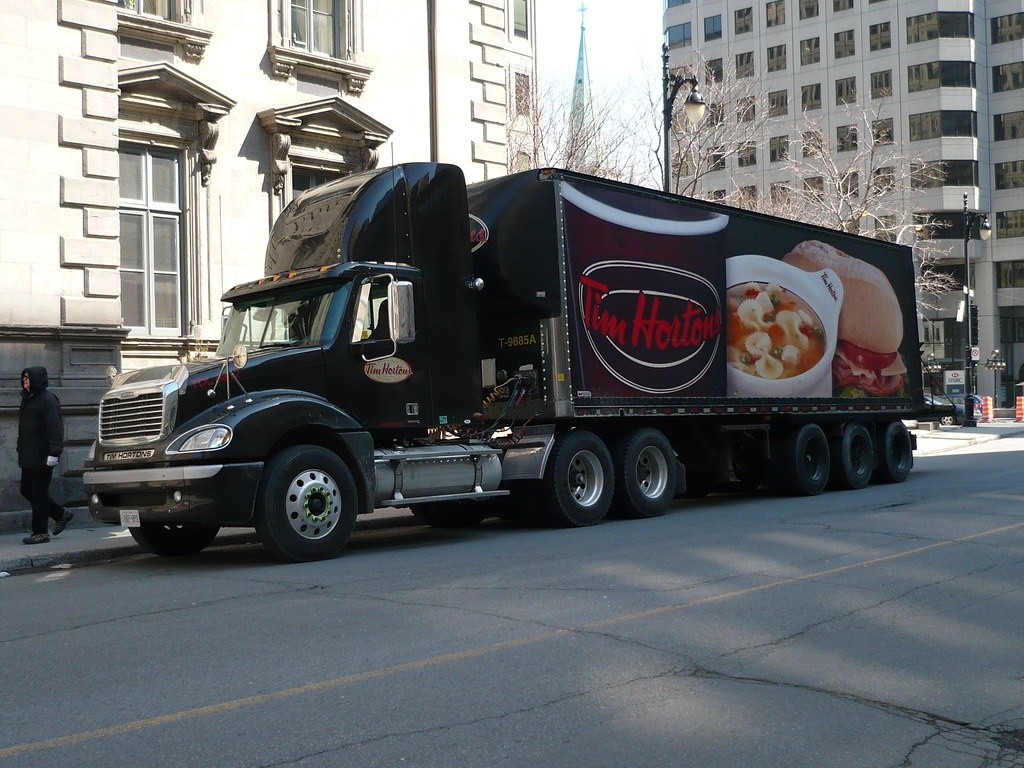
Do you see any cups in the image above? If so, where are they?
[726,256,845,397]
[559,180,729,397]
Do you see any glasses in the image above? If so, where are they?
[24,375,29,379]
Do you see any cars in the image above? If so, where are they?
[939,394,984,417]
[922,394,983,427]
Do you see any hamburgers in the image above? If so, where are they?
[783,240,908,398]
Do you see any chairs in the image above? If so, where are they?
[366,299,391,340]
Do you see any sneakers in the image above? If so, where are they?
[22,533,50,544]
[53,507,74,535]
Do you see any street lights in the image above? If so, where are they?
[660,42,707,196]
[961,191,992,430]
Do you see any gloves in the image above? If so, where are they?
[46,455,59,467]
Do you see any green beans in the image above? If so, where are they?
[739,294,822,364]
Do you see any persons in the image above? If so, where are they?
[16,366,74,543]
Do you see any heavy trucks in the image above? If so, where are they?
[84,163,958,564]
